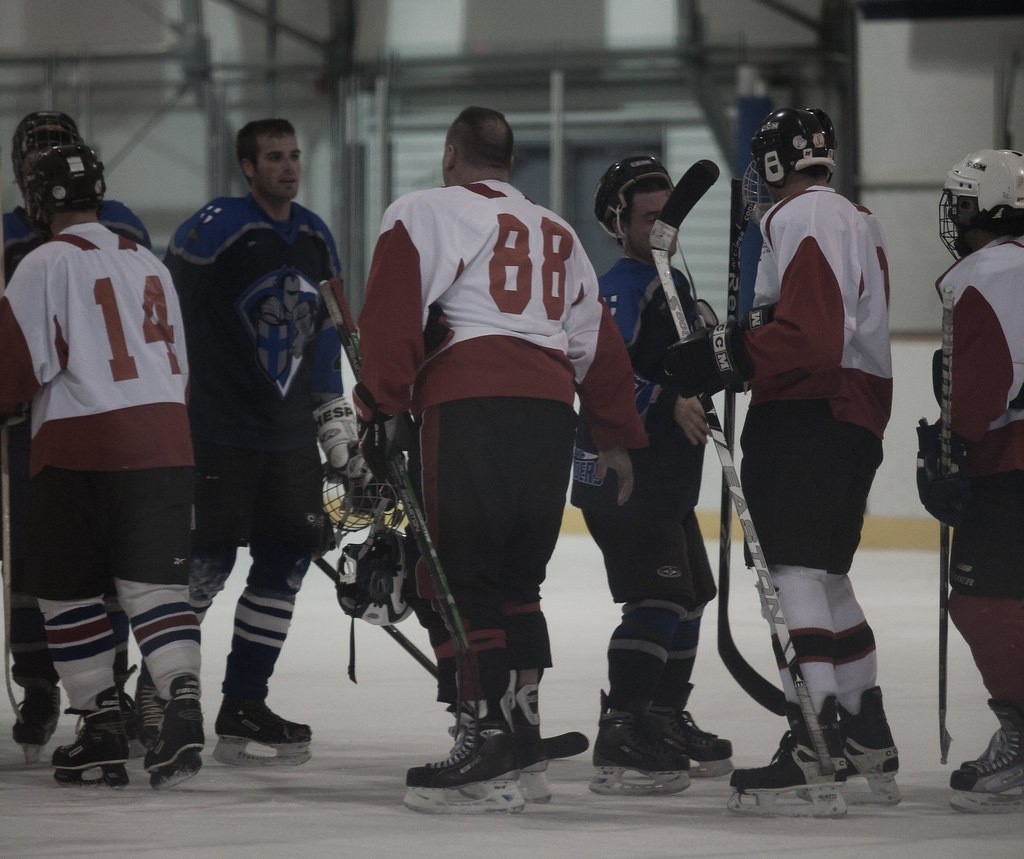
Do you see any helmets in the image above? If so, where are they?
[741,106,838,227]
[13,109,81,197]
[593,153,676,243]
[937,149,1024,263]
[25,138,107,230]
[336,525,417,627]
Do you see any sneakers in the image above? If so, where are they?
[404,677,553,814]
[12,660,61,764]
[589,684,690,799]
[144,673,206,790]
[134,654,165,751]
[947,700,1024,813]
[836,685,901,806]
[730,694,848,819]
[114,666,149,758]
[50,684,134,786]
[213,684,312,769]
[652,679,734,778]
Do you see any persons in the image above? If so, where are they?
[917,150,1024,814]
[0,112,153,765]
[134,118,374,767]
[352,107,650,813]
[571,155,733,795]
[0,144,205,790]
[651,108,899,817]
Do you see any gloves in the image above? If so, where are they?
[916,418,980,530]
[352,382,400,460]
[314,395,371,481]
[664,319,752,398]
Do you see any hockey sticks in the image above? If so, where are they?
[315,275,484,750]
[314,556,592,761]
[714,175,788,717]
[647,158,839,777]
[935,283,956,766]
[2,421,26,724]
